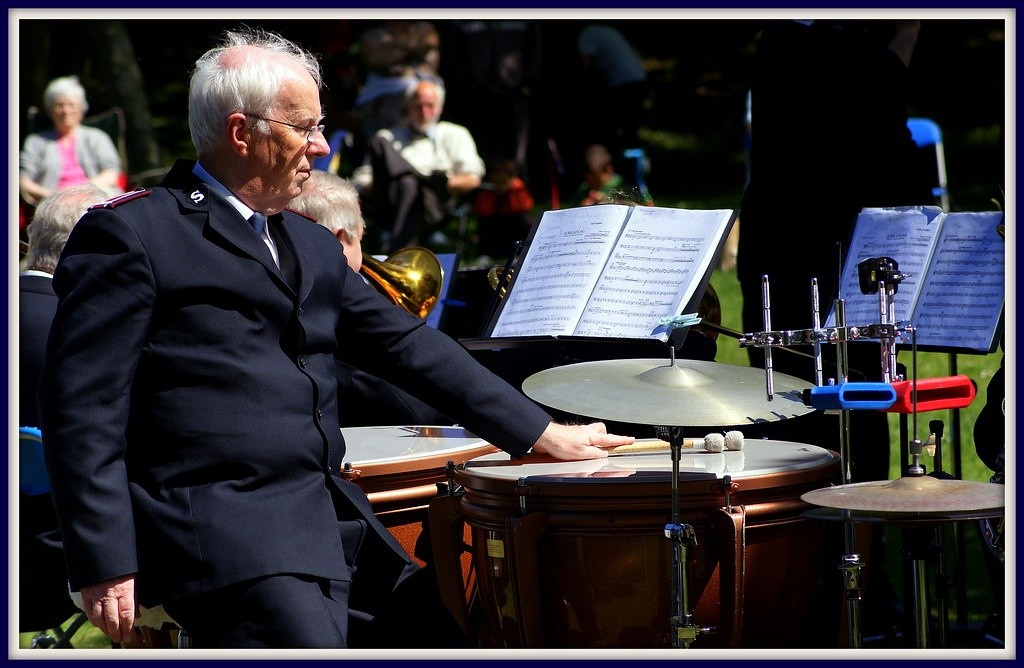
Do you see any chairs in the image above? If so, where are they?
[19,426,121,649]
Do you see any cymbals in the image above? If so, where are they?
[520,358,820,427]
[798,476,1005,514]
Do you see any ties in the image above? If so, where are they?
[248,212,264,237]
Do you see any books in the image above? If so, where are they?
[485,205,740,350]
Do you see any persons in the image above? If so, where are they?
[19,76,121,210]
[693,20,929,486]
[43,25,636,649]
[820,206,1005,354]
[364,20,622,251]
[19,182,112,431]
[284,168,452,427]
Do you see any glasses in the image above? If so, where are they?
[226,111,325,144]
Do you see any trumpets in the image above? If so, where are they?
[358,246,445,319]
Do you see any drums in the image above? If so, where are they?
[450,436,844,649]
[338,425,502,648]
